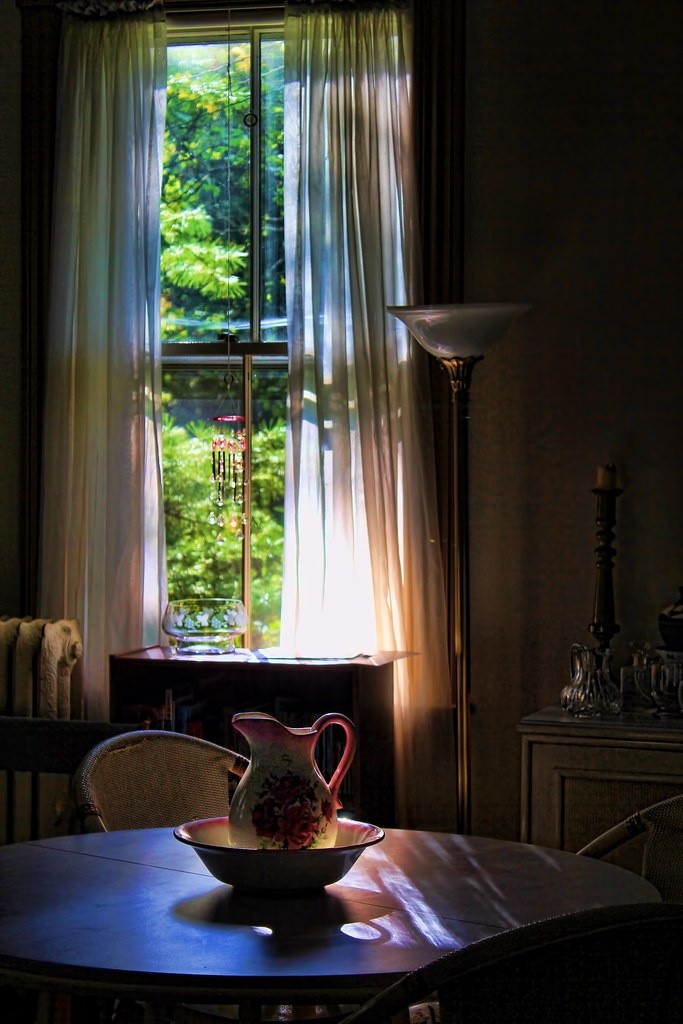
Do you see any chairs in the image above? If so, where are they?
[342,794,683,1024]
[70,729,249,833]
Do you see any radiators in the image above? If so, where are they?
[0,617,83,844]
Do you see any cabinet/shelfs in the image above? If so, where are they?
[517,707,683,876]
[108,645,421,828]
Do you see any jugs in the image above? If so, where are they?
[633,647,683,719]
[230,711,356,849]
[560,642,624,719]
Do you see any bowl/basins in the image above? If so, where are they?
[174,814,386,891]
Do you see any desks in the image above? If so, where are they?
[0,828,660,1024]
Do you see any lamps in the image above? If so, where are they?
[388,302,536,834]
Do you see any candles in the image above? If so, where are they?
[597,463,617,489]
